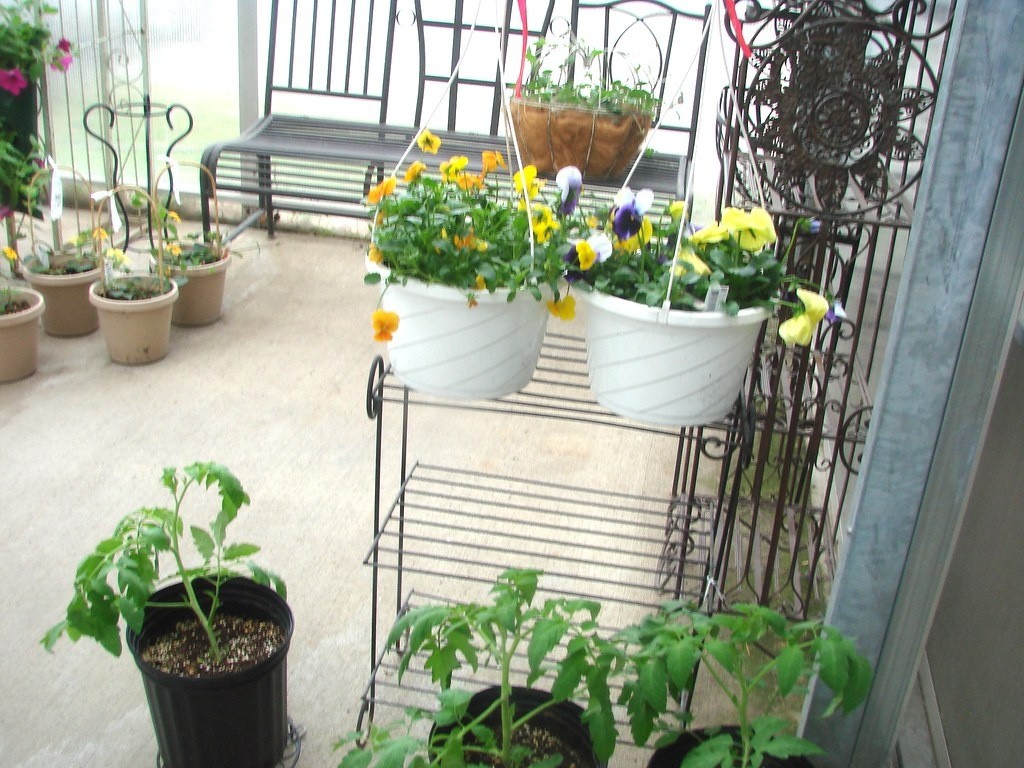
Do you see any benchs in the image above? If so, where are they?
[195,0,719,275]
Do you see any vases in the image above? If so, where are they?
[22,248,108,339]
[0,285,45,385]
[564,250,781,429]
[87,272,180,367]
[363,217,559,401]
[148,238,233,328]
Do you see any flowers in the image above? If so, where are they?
[0,22,76,224]
[91,185,173,300]
[152,160,230,273]
[0,241,25,316]
[360,127,600,346]
[27,164,101,277]
[549,162,851,350]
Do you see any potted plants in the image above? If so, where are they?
[36,459,304,768]
[330,567,629,768]
[609,599,880,768]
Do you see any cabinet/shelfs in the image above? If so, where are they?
[352,329,880,758]
